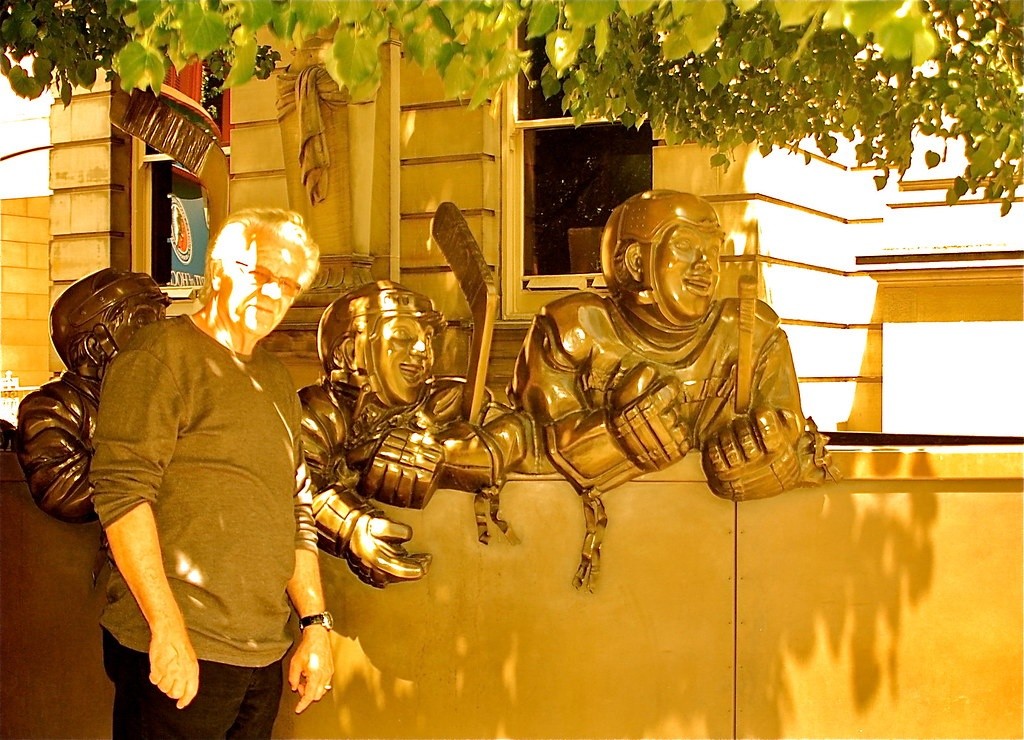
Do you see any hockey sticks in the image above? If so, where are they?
[426,203,503,430]
[735,275,757,415]
[97,74,231,291]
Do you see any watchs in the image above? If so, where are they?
[299,610,333,632]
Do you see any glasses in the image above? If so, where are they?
[223,257,304,300]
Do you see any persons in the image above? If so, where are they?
[88,209,340,740]
[13,264,172,523]
[293,278,527,592]
[506,185,830,503]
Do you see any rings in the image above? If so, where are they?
[324,685,332,690]
